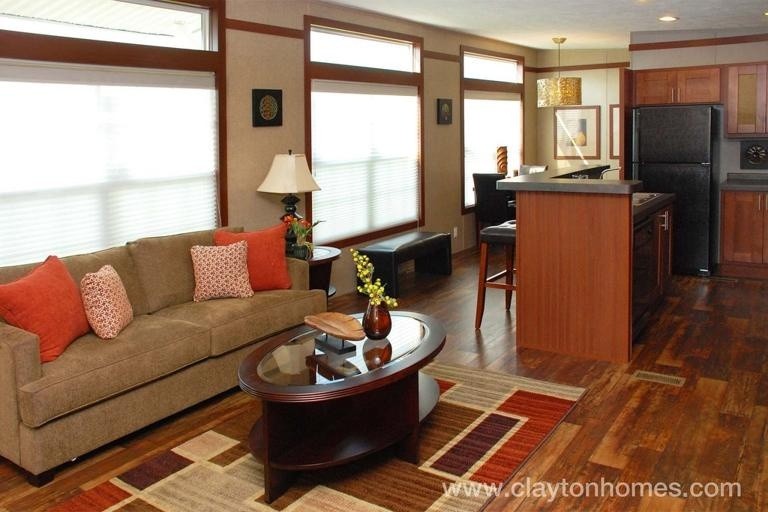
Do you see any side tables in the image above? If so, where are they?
[306,244,342,300]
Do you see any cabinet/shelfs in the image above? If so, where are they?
[633,67,723,107]
[719,189,767,280]
[722,61,767,139]
[634,203,673,341]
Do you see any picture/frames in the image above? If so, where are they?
[553,103,620,160]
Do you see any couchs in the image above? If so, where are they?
[0,222,330,489]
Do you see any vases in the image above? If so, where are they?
[359,297,393,342]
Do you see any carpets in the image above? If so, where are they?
[48,359,590,510]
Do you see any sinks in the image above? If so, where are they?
[633,192,662,206]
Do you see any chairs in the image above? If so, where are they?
[472,170,519,225]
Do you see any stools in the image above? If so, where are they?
[475,215,519,330]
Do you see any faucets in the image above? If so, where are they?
[599,167,622,179]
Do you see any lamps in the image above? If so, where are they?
[255,146,323,252]
[536,35,583,108]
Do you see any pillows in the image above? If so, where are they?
[0,252,135,366]
[187,224,295,302]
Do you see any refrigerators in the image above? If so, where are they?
[628,105,720,277]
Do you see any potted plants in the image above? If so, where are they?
[289,218,326,258]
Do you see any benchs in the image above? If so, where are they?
[355,228,453,300]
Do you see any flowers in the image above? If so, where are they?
[349,247,402,309]
